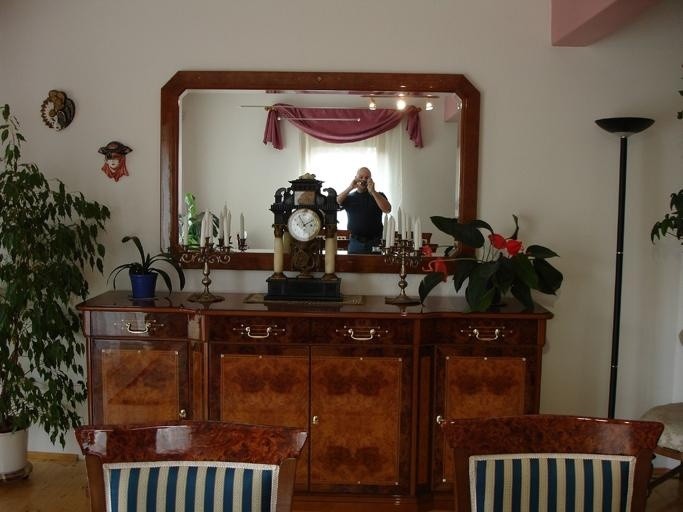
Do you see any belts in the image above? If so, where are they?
[350,233,383,242]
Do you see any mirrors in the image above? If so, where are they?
[158,69,482,271]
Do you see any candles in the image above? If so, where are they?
[181,200,231,248]
[386,206,423,252]
[382,214,387,240]
[239,212,245,240]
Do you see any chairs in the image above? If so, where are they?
[433,409,664,510]
[67,424,310,510]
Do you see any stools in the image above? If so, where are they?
[636,399,682,498]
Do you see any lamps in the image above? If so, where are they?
[346,86,440,113]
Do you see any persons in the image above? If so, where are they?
[183,192,201,245]
[336,166,391,255]
[97,141,133,182]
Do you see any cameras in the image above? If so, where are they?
[359,179,367,185]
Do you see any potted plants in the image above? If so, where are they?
[104,234,184,302]
[0,96,110,483]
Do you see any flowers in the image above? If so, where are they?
[413,208,564,310]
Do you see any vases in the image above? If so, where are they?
[464,281,501,311]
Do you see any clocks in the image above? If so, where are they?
[266,171,342,303]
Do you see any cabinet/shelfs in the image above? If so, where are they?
[414,315,539,493]
[85,312,205,424]
[205,315,414,494]
